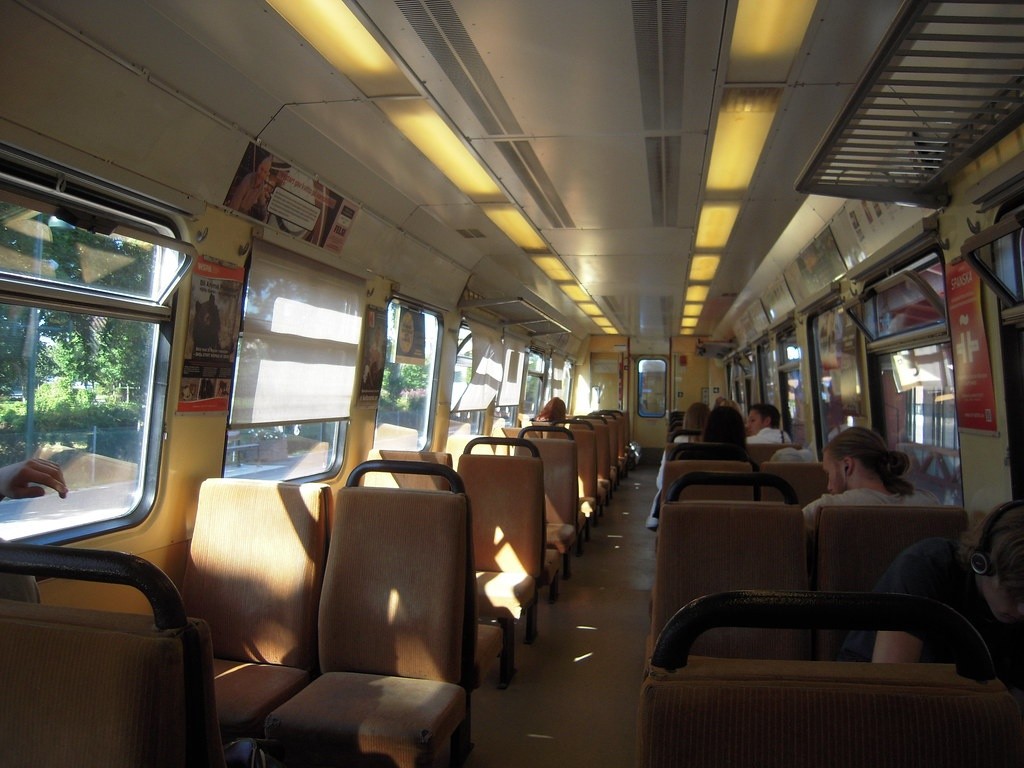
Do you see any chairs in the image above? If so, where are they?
[647,469,811,665]
[263,460,477,768]
[817,502,970,663]
[759,459,829,510]
[364,390,629,689]
[640,590,1024,768]
[189,476,333,742]
[661,444,760,501]
[0,544,221,768]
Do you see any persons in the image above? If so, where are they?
[645,378,942,554]
[536,397,566,423]
[0,458,68,502]
[229,157,272,225]
[838,500,1024,705]
